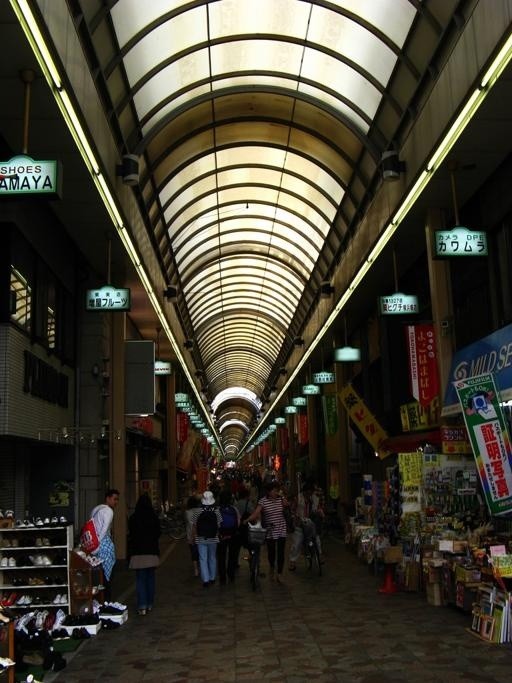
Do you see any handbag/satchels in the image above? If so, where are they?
[240,513,256,550]
[80,520,99,556]
[283,505,296,534]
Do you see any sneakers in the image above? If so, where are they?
[42,538,51,546]
[0,592,67,606]
[14,610,69,672]
[12,539,19,547]
[11,575,63,585]
[36,538,43,546]
[1,554,51,567]
[92,599,128,629]
[92,586,99,595]
[62,612,100,640]
[0,539,11,547]
[135,607,151,615]
[0,509,67,529]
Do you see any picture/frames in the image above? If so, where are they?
[472,582,511,644]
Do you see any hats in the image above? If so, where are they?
[200,490,215,506]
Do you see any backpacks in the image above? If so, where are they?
[219,505,239,537]
[196,507,217,540]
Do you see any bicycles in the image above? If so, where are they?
[242,517,275,591]
[154,501,188,541]
[290,510,327,575]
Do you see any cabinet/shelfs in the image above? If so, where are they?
[69,550,105,613]
[0,526,73,617]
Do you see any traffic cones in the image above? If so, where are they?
[379,563,401,593]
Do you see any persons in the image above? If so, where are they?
[182,464,324,590]
[125,492,161,614]
[81,488,120,616]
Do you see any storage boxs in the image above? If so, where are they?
[380,539,481,613]
[59,607,128,634]
